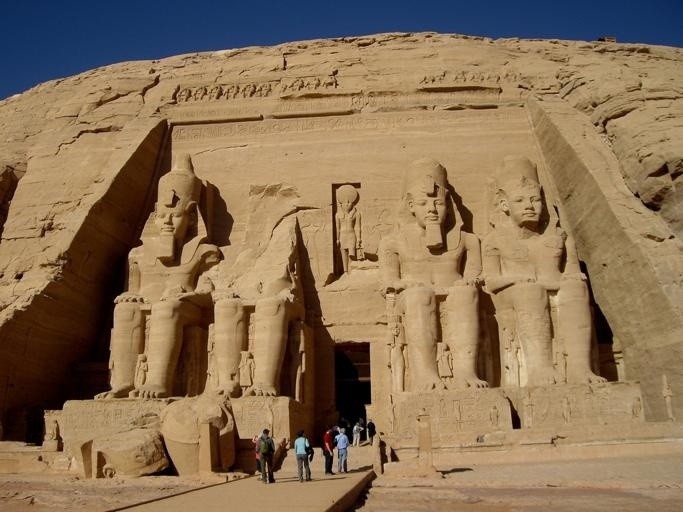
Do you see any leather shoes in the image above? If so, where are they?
[299,479,312,483]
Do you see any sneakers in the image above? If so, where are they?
[325,470,347,475]
[264,480,275,483]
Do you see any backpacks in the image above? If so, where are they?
[259,437,270,455]
[353,426,359,433]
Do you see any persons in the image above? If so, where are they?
[335,200,360,272]
[214,297,305,396]
[252,418,376,484]
[94,189,220,399]
[483,179,608,386]
[238,351,256,394]
[379,179,482,390]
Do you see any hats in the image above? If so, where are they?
[297,430,304,437]
